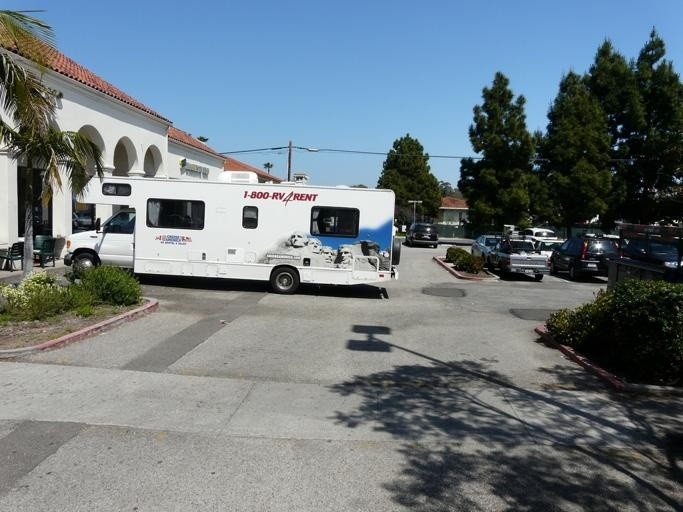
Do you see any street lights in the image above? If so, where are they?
[407,200,422,224]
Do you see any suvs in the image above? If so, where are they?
[405,222,438,249]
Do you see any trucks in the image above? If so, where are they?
[61,176,403,294]
[605,222,682,285]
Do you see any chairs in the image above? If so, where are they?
[0,234,64,272]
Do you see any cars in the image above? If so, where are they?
[71,210,91,230]
[469,228,623,280]
